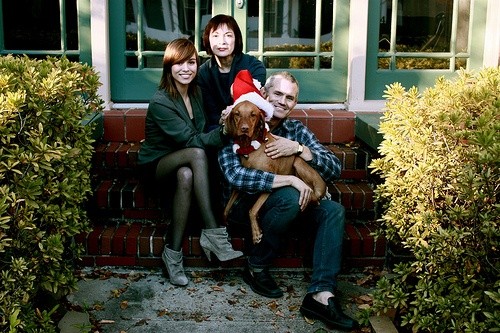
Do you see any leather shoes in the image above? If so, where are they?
[241,261,284,298]
[299,293,359,331]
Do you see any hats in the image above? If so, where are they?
[233,69,274,122]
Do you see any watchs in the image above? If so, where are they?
[297,142,303,156]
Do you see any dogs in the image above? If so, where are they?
[222,101,327,247]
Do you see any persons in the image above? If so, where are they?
[217,69,360,331]
[134,38,243,287]
[195,15,267,134]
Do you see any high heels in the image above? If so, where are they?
[162,244,189,286]
[200,225,244,262]
